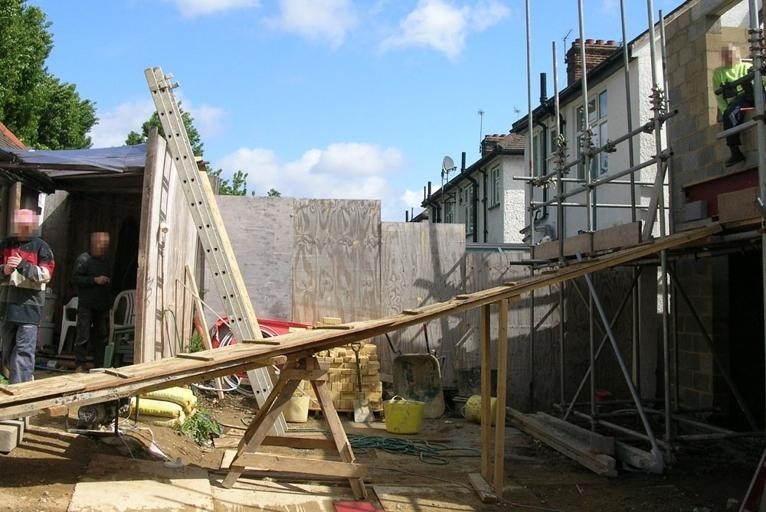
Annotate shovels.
[351,343,376,422]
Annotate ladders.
[146,67,288,437]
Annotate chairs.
[57,288,136,368]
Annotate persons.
[0,202,56,385]
[72,225,114,373]
[713,42,766,168]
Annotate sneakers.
[724,152,746,168]
